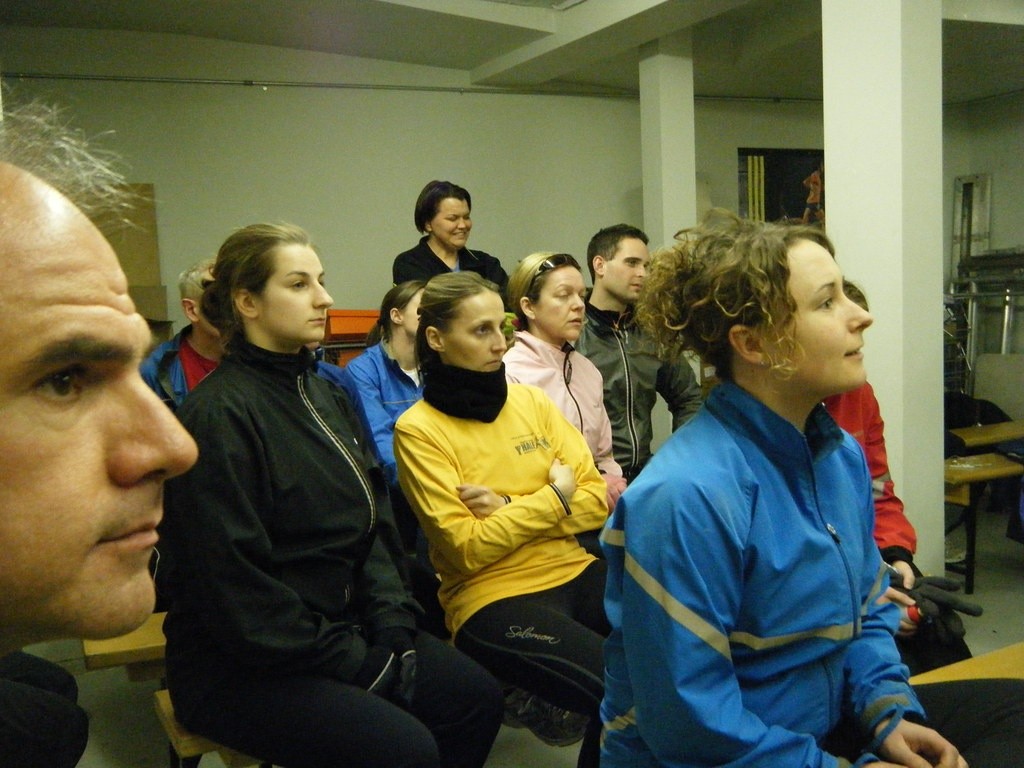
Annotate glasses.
[524,254,580,297]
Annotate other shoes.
[500,670,593,748]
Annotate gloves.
[368,648,418,703]
[882,562,984,638]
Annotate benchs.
[81,612,264,767]
[943,419,1024,595]
[907,639,1023,686]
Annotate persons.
[0,159,974,768]
[393,180,511,292]
[574,223,704,487]
[345,280,429,555]
[502,252,627,513]
